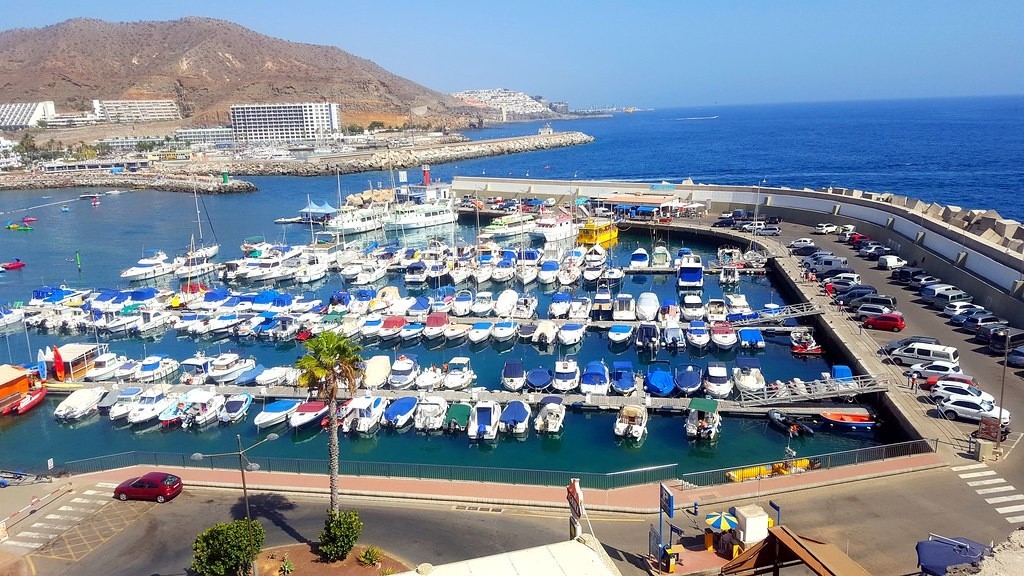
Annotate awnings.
[298,201,338,221]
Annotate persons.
[800,268,817,288]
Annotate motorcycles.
[971,428,1015,442]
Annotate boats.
[727,456,826,485]
[3,197,880,452]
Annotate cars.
[114,471,183,504]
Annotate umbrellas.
[705,510,738,553]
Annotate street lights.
[190,431,280,576]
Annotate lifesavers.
[614,427,622,435]
[632,430,640,437]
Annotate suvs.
[710,206,1024,425]
